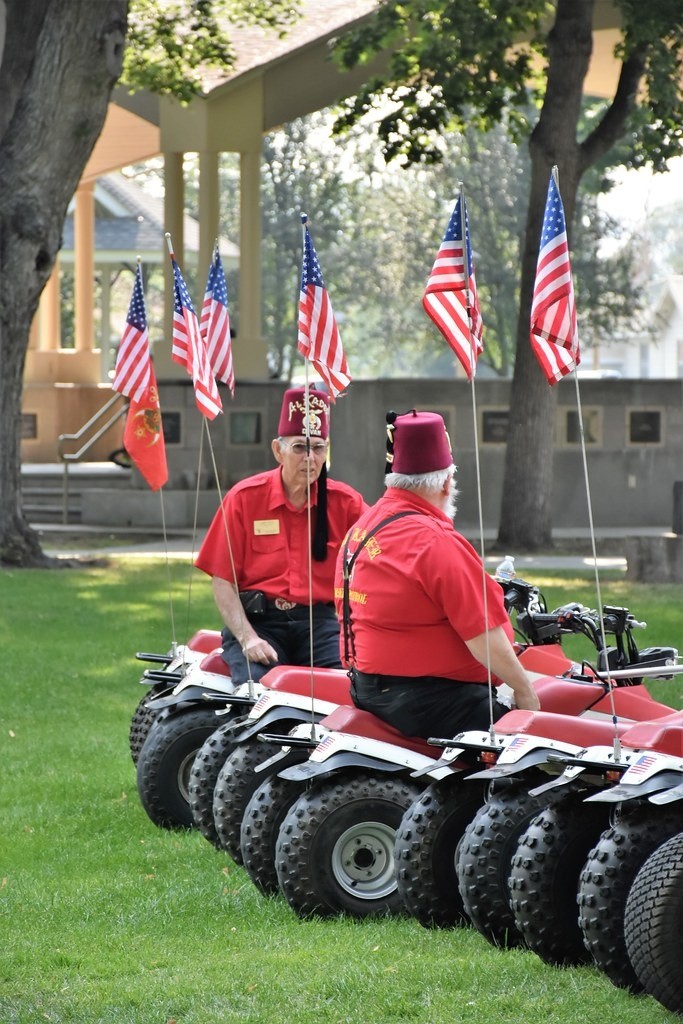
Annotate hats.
[278,382,331,561]
[382,408,453,474]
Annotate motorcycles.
[129,573,683,1015]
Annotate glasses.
[279,437,328,455]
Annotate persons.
[194,388,372,690]
[333,409,540,742]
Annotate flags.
[422,195,483,383]
[171,251,223,420]
[113,264,168,491]
[530,171,580,386]
[297,216,351,398]
[198,246,235,397]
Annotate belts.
[266,596,304,610]
